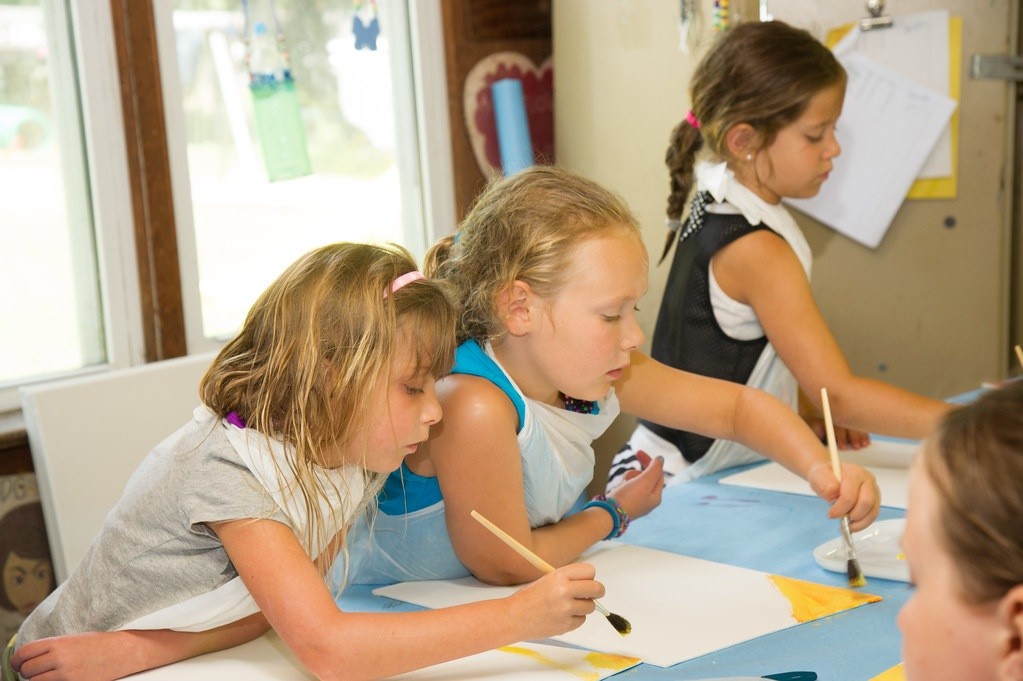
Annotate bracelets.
[583,495,630,541]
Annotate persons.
[608,21,960,493]
[0,242,603,681]
[323,166,881,593]
[897,379,1022,681]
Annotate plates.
[812,517,911,584]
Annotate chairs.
[21,351,224,584]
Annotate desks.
[110,385,1000,681]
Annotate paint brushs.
[469,510,632,637]
[820,386,867,588]
